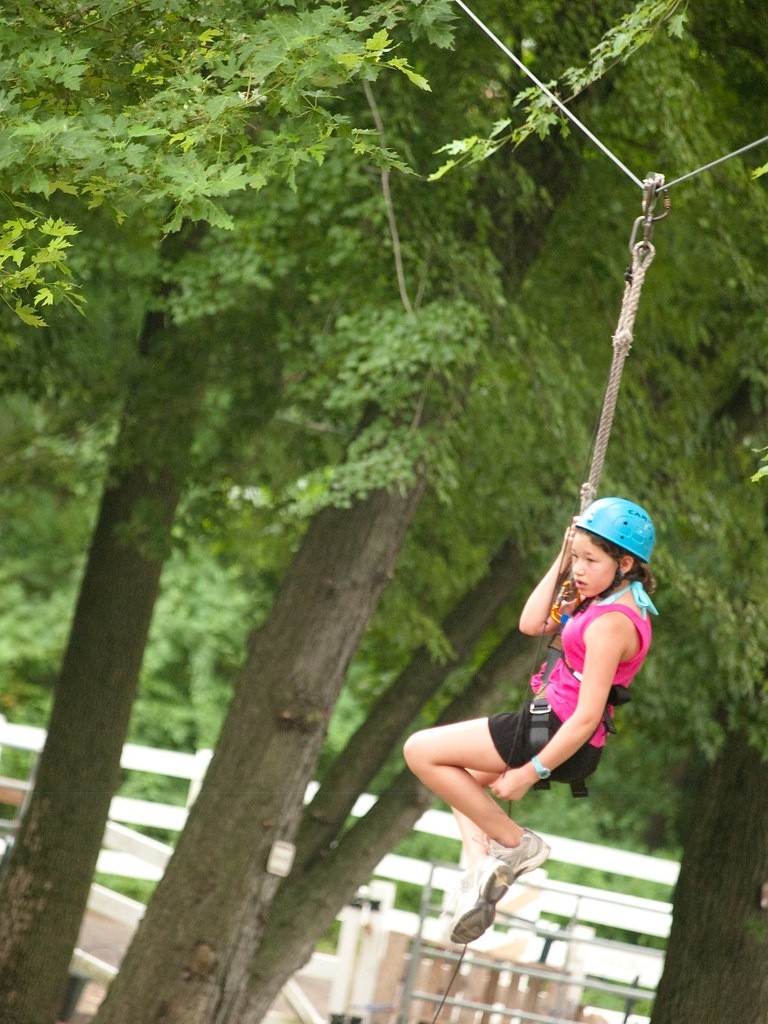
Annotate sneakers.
[488,825,550,878]
[450,858,514,945]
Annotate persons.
[402,496,658,944]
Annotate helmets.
[575,497,655,563]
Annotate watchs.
[532,756,551,779]
